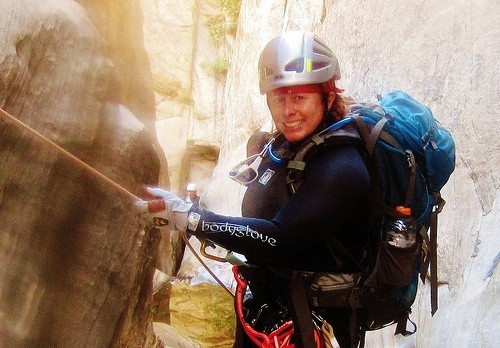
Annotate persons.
[134,31,380,348]
[182,183,201,260]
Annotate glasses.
[228,138,275,184]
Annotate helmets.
[258,31,340,94]
[187,184,197,191]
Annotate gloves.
[132,187,189,239]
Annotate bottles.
[388,207,417,247]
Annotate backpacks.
[247,92,457,331]
